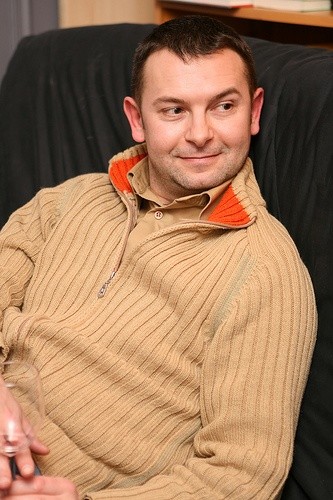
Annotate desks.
[153,0,333,50]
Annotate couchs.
[0,22,333,500]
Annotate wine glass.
[0,361,42,480]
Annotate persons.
[0,15,319,500]
